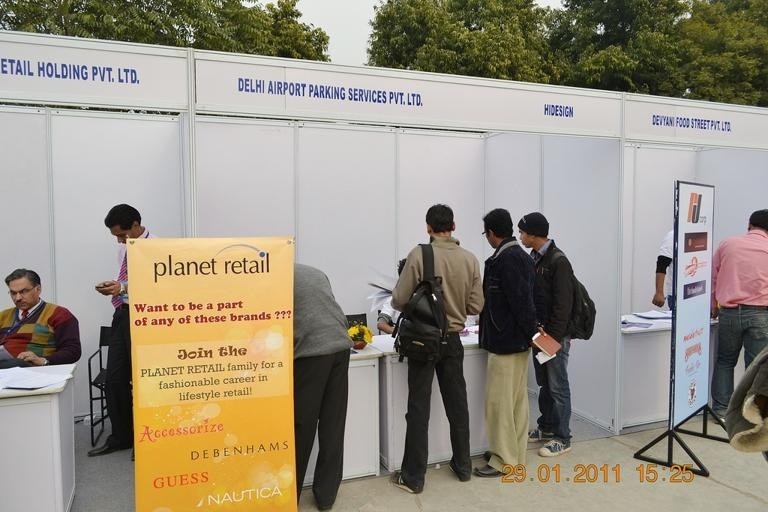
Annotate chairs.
[88,326,112,447]
[377,309,387,335]
[343,313,368,331]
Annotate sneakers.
[527,429,553,443]
[392,471,424,494]
[449,460,471,481]
[539,438,571,456]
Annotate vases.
[353,341,367,350]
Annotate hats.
[518,212,549,236]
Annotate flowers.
[347,322,374,345]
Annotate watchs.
[119,280,126,298]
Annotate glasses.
[481,231,488,238]
[8,287,35,296]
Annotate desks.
[295,343,383,488]
[1,365,77,512]
[620,308,717,432]
[368,328,490,474]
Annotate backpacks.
[547,252,596,341]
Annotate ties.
[111,256,127,310]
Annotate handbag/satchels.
[394,279,447,363]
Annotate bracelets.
[41,356,49,366]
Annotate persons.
[652,230,674,312]
[376,258,408,334]
[87,204,162,461]
[294,261,353,511]
[473,209,546,477]
[0,269,81,367]
[390,203,485,493]
[710,209,768,423]
[518,212,575,458]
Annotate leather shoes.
[474,464,501,478]
[483,451,491,462]
[87,444,118,456]
